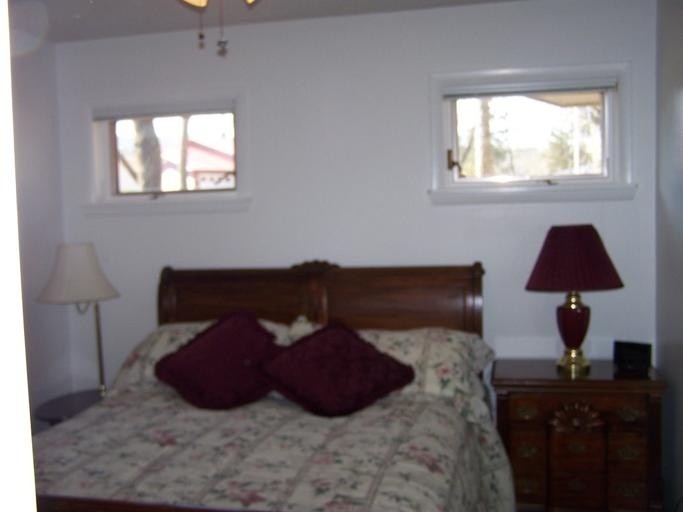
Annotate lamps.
[526,224,623,373]
[37,241,121,392]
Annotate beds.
[32,261,483,512]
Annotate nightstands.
[34,390,107,424]
[491,357,667,512]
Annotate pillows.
[287,315,492,415]
[152,312,281,412]
[258,316,415,417]
[111,319,287,402]
